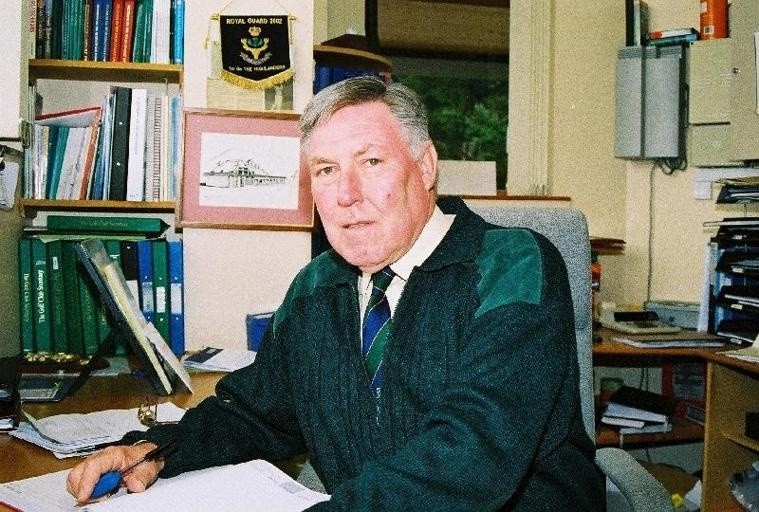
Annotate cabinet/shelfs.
[698,348,759,512]
[703,221,759,345]
[23,56,185,373]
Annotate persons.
[65,74,608,512]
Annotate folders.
[152,241,168,344]
[121,241,139,309]
[168,240,183,355]
[137,240,153,324]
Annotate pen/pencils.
[73,438,177,508]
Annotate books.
[644,27,698,45]
[601,384,680,437]
[25,0,184,63]
[26,86,183,201]
[24,205,195,397]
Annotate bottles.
[626,1,649,47]
[699,0,728,40]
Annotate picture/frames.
[174,108,315,233]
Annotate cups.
[599,376,624,409]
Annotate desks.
[1,371,331,512]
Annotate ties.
[362,266,395,399]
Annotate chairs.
[314,195,677,512]
